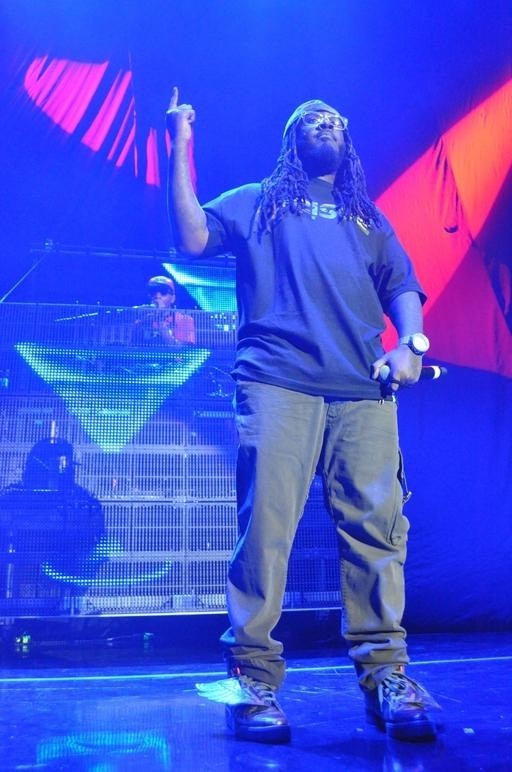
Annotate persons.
[132,276,197,362]
[164,87,444,742]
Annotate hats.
[281,100,341,139]
[148,276,175,294]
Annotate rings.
[390,377,404,386]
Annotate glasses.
[149,287,171,296]
[292,111,350,131]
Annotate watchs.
[397,331,431,356]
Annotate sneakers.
[364,667,438,740]
[224,675,291,742]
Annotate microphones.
[131,304,156,313]
[378,363,450,384]
